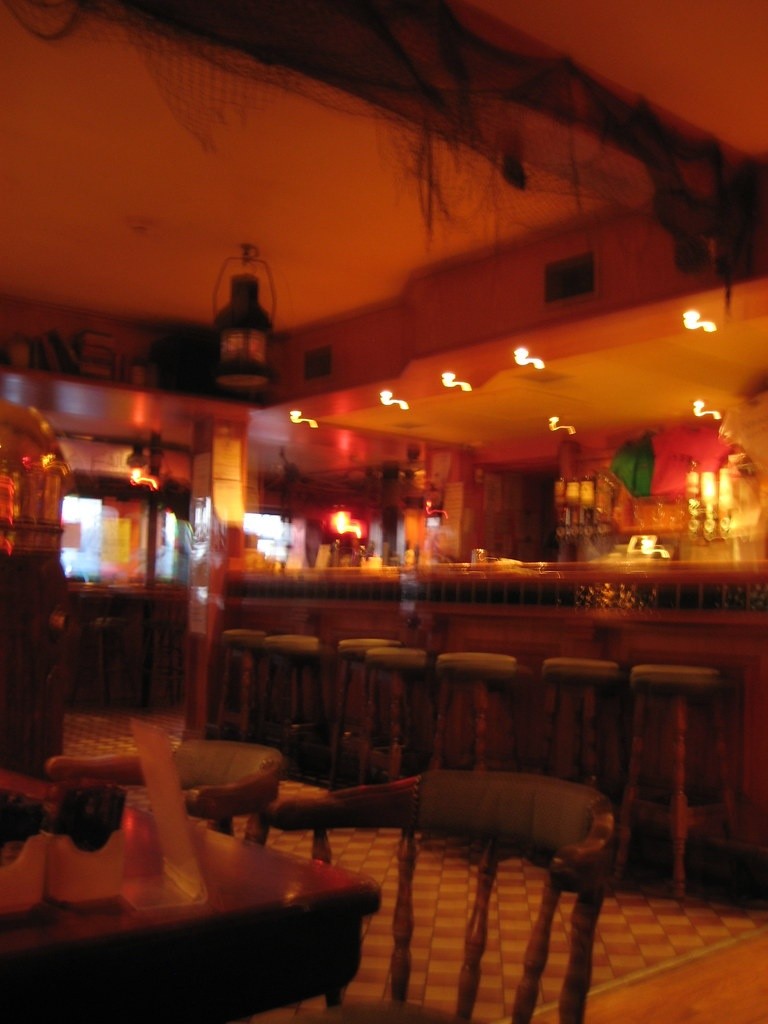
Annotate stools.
[421,651,516,855]
[613,665,740,900]
[328,639,402,789]
[257,634,328,784]
[538,657,620,789]
[215,629,267,742]
[68,614,185,713]
[359,648,428,784]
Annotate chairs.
[267,768,618,1024]
[44,739,284,846]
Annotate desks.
[0,768,381,1024]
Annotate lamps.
[214,243,280,395]
